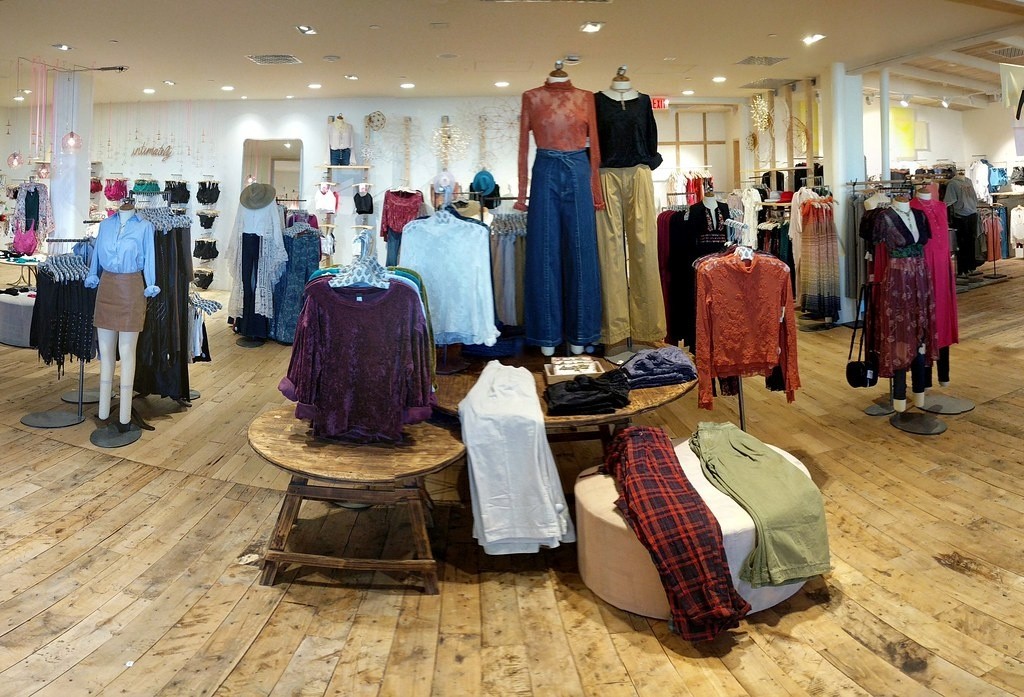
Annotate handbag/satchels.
[846,360,875,388]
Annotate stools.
[575,443,812,622]
[0,291,36,347]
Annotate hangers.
[185,288,223,314]
[661,167,839,273]
[38,174,222,282]
[286,178,528,289]
[24,175,38,186]
[971,156,1024,225]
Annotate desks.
[248,404,467,596]
[0,259,39,287]
[432,340,700,454]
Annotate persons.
[84,204,161,432]
[872,187,959,413]
[944,172,978,275]
[513,61,606,358]
[224,183,289,340]
[585,65,668,344]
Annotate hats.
[240,183,276,209]
[434,172,455,193]
[472,171,495,196]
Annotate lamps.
[969,97,976,106]
[899,94,914,107]
[942,97,952,109]
[35,69,50,179]
[62,63,82,153]
[7,57,23,169]
